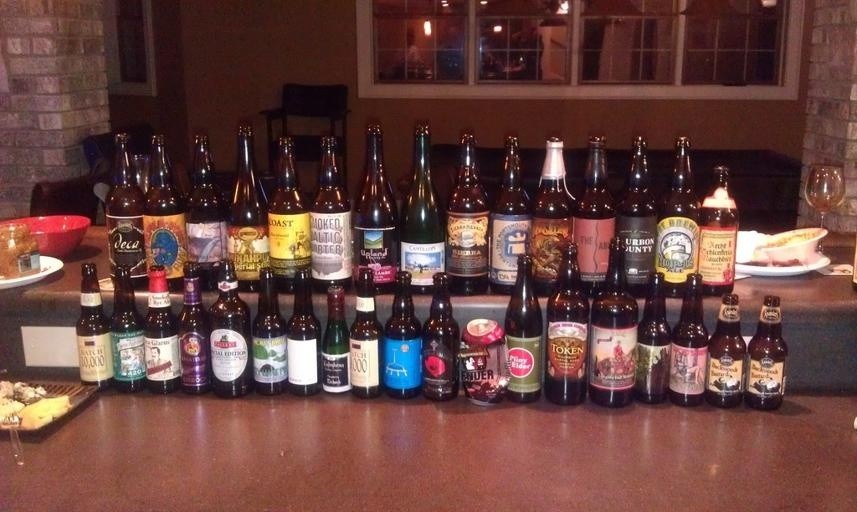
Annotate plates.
[734,253,830,278]
[0,256,65,289]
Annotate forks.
[3,412,25,469]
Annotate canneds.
[458,318,514,408]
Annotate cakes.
[0,379,69,431]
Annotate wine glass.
[804,163,843,255]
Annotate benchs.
[395,142,798,233]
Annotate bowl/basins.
[754,225,828,263]
[0,215,92,259]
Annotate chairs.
[258,83,350,186]
[26,178,101,225]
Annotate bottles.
[107,134,742,291]
[75,236,786,409]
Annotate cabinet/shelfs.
[104,1,179,99]
